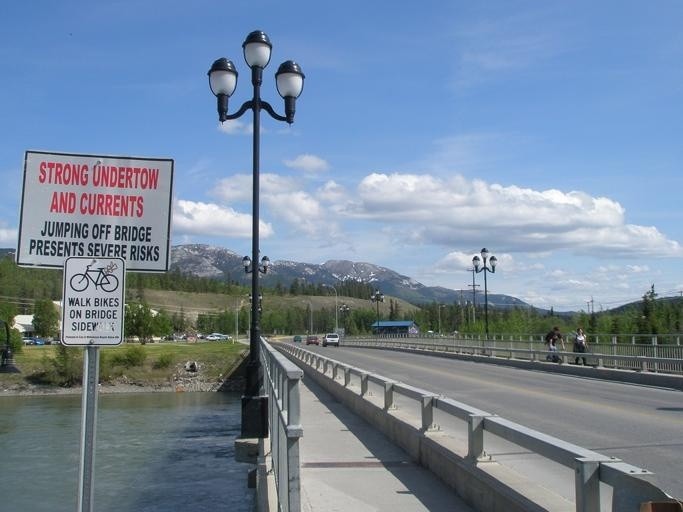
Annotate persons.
[546,326,566,362]
[572,328,589,366]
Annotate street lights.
[472,248,496,340]
[370,289,383,334]
[206,31,304,438]
[339,303,350,335]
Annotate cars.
[22,337,46,345]
[306,336,318,345]
[322,333,339,347]
[161,332,229,342]
[293,336,301,342]
